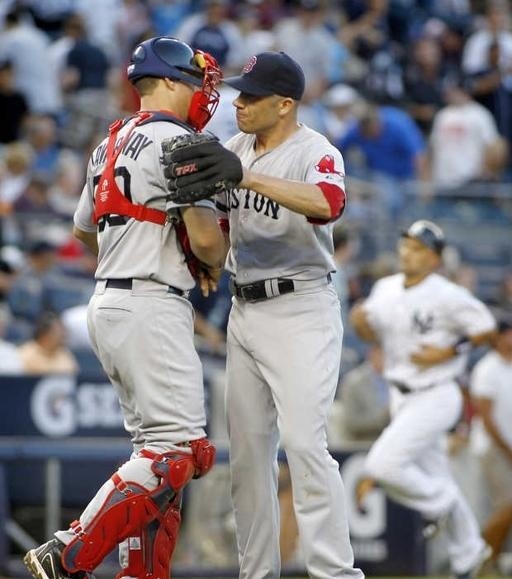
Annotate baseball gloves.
[159,131,243,204]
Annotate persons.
[467,309,511,578]
[0,0,512,451]
[352,217,496,579]
[171,49,363,579]
[22,38,230,579]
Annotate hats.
[220,52,304,100]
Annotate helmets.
[400,220,444,256]
[128,37,224,132]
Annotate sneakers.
[24,539,94,578]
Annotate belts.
[228,276,293,304]
[394,382,411,393]
[104,279,185,295]
